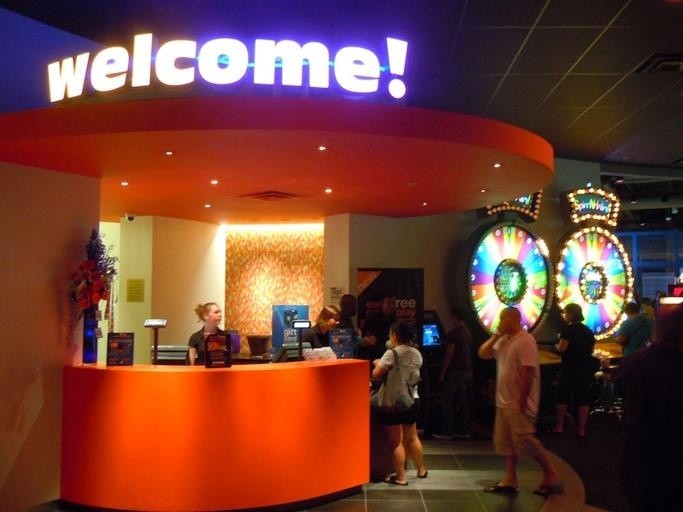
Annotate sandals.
[534,482,566,497]
[482,482,519,494]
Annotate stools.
[593,364,626,423]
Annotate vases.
[82,303,98,364]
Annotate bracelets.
[492,330,500,339]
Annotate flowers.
[68,227,120,339]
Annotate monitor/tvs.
[151,346,191,365]
[422,323,441,346]
[272,342,312,363]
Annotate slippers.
[418,470,429,480]
[382,473,410,486]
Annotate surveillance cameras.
[126,214,135,221]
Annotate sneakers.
[433,431,473,441]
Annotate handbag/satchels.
[377,349,412,411]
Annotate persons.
[478,305,564,495]
[337,293,360,350]
[305,304,338,349]
[545,303,597,438]
[186,303,231,365]
[371,322,427,485]
[363,296,397,358]
[613,290,667,358]
[431,305,475,441]
